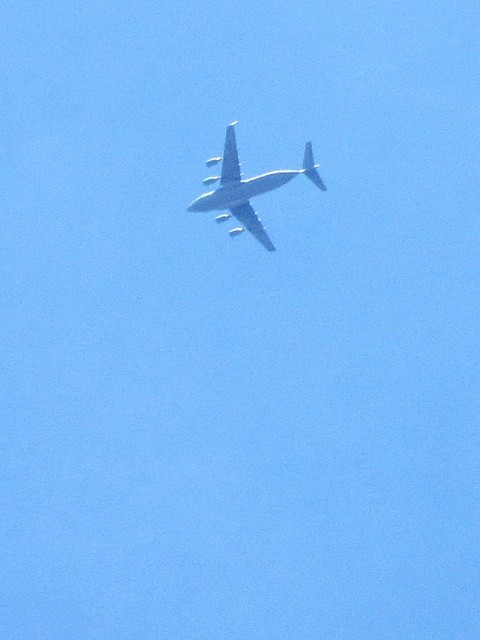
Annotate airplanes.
[187,119,331,253]
